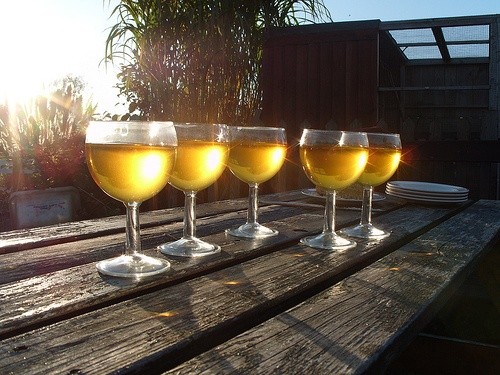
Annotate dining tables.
[1,187,500,375]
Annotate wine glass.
[225,126,287,238]
[157,122,231,258]
[299,128,369,251]
[340,133,402,240]
[85,121,178,278]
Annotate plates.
[302,189,386,202]
[384,181,470,203]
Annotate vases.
[9,186,82,228]
